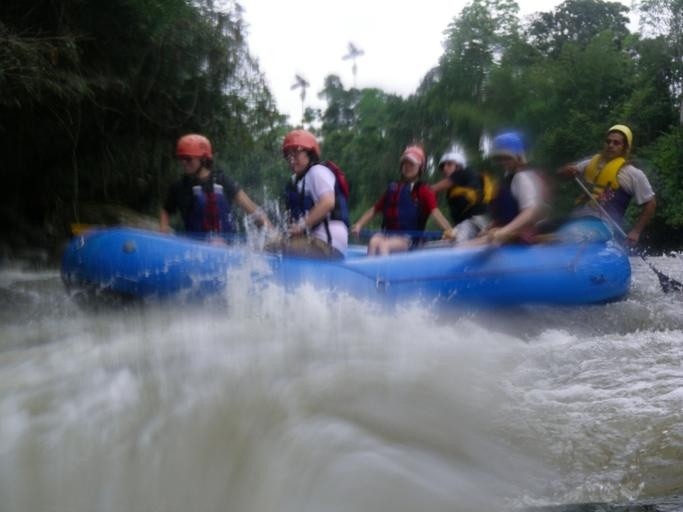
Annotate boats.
[59,215,632,325]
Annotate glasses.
[604,138,620,145]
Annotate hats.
[399,146,423,165]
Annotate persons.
[462,133,553,248]
[160,134,280,248]
[431,151,494,242]
[349,145,460,258]
[553,123,656,248]
[260,129,352,258]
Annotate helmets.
[607,124,632,147]
[483,132,525,161]
[283,130,318,156]
[175,134,211,160]
[437,153,466,171]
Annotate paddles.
[574,176,683,296]
